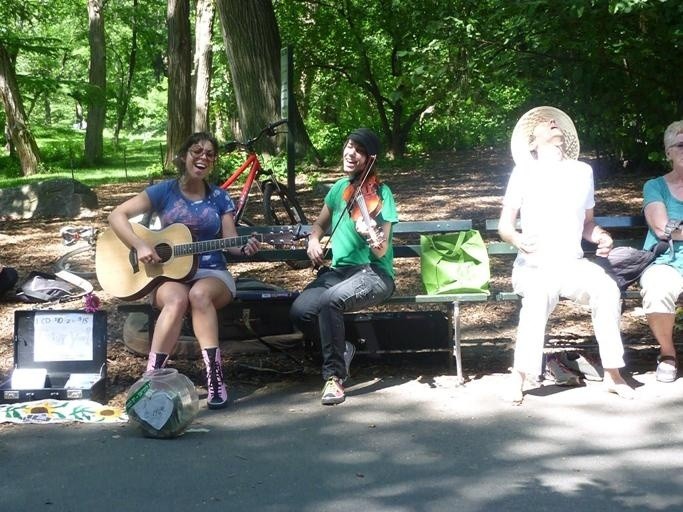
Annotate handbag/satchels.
[419,229,490,296]
[592,218,681,291]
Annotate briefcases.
[1,309,107,404]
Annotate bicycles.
[217,117,312,271]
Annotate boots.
[147,351,169,372]
[202,346,228,409]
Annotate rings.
[147,257,151,262]
[144,261,148,264]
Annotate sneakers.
[541,353,580,386]
[321,375,346,404]
[343,341,357,385]
[556,350,603,381]
[654,354,678,382]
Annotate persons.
[497,116,639,408]
[638,119,683,383]
[287,127,400,406]
[107,131,262,409]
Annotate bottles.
[123,367,202,439]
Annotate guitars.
[95,222,294,300]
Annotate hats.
[349,127,379,153]
[510,106,580,164]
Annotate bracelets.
[598,231,610,237]
[239,244,246,257]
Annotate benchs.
[487,214,681,381]
[116,216,492,386]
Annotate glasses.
[669,142,682,147]
[188,144,219,161]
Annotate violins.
[343,175,386,249]
